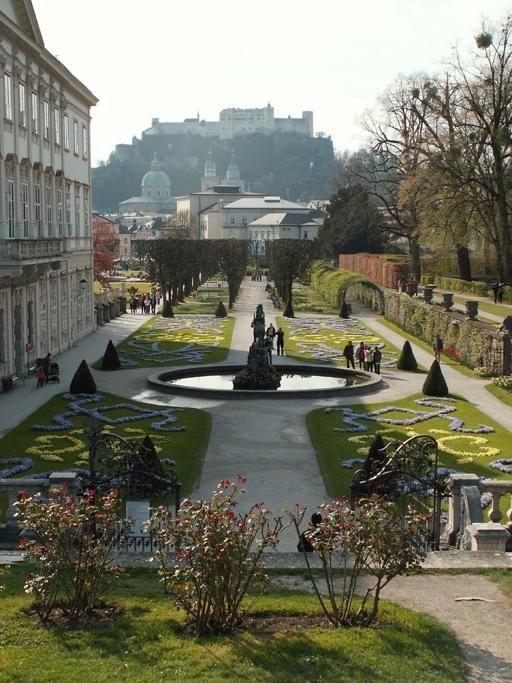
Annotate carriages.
[128,292,145,314]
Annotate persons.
[272,326,285,355]
[433,335,443,362]
[36,351,59,388]
[492,281,499,303]
[297,512,326,552]
[266,322,275,350]
[129,288,162,313]
[265,337,276,364]
[343,340,382,374]
[396,272,419,298]
[497,284,504,303]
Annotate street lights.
[151,286,157,315]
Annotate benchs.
[2,373,26,391]
[27,359,40,377]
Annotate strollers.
[46,362,62,384]
